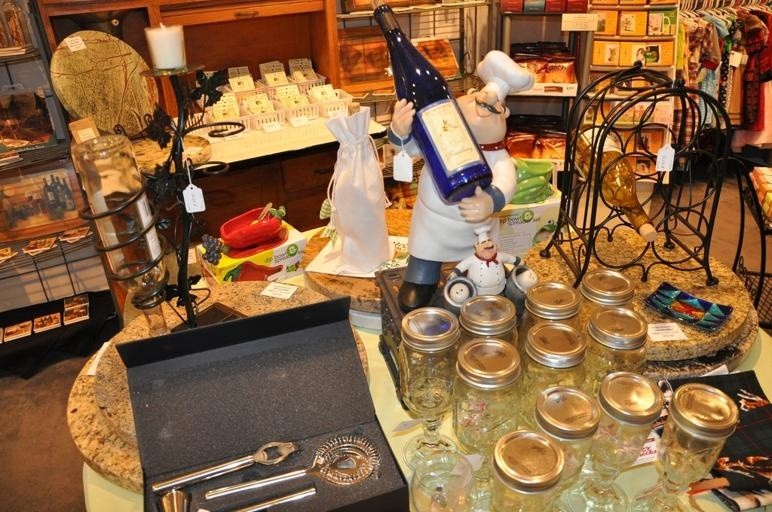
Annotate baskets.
[270,93,319,124]
[242,98,288,130]
[172,113,217,139]
[226,81,266,106]
[197,87,234,108]
[288,71,327,95]
[308,88,354,120]
[206,103,253,134]
[256,76,300,100]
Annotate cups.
[412,450,479,511]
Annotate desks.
[82,219,772,511]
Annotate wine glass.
[521,320,588,425]
[398,306,459,468]
[519,282,580,355]
[629,383,738,512]
[586,306,648,390]
[532,387,600,496]
[567,372,662,512]
[490,429,563,512]
[456,294,519,347]
[451,337,522,453]
[579,268,633,335]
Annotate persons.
[387,51,534,313]
[446,225,527,297]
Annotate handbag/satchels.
[317,105,393,273]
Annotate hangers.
[678,0,772,42]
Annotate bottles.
[569,125,656,242]
[41,174,74,212]
[72,135,171,340]
[371,1,493,206]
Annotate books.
[625,156,660,181]
[1,246,14,261]
[585,100,671,125]
[592,40,675,67]
[59,231,95,244]
[62,226,91,242]
[23,244,60,256]
[608,129,664,154]
[593,8,676,36]
[24,237,56,251]
[589,72,668,95]
[591,0,679,6]
[1,252,20,266]
[0,293,89,344]
